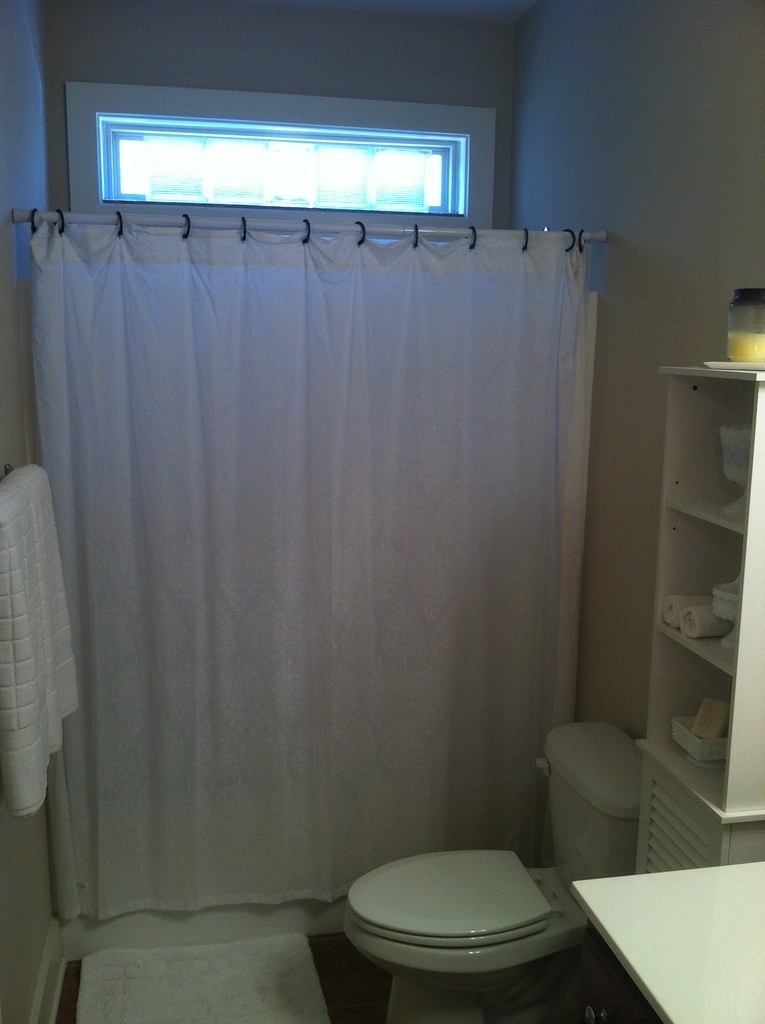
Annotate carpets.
[75,932,333,1024]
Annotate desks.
[571,861,765,1024]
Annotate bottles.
[727,289,765,361]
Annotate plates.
[702,361,764,370]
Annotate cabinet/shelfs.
[633,366,765,874]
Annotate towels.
[663,594,736,638]
[0,463,79,821]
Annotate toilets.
[342,722,643,1023]
